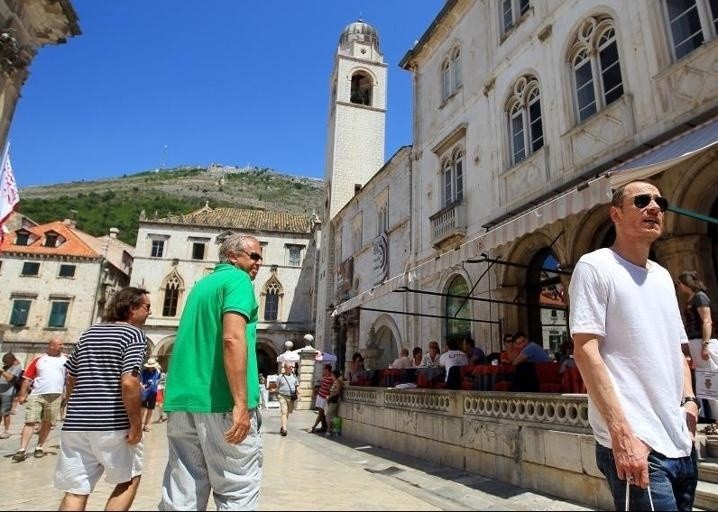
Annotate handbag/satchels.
[289,392,298,400]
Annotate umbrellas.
[277,345,337,362]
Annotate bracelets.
[702,341,709,346]
[681,397,701,412]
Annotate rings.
[626,475,634,482]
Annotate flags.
[0,153,20,253]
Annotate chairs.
[350,360,587,394]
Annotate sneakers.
[12,450,27,461]
[280,426,337,437]
[0,431,12,439]
[34,446,44,458]
[143,415,167,432]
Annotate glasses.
[139,303,151,312]
[235,249,262,262]
[616,194,669,213]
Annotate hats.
[143,358,159,368]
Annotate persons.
[0,338,269,461]
[348,332,576,392]
[324,371,342,436]
[53,287,152,512]
[308,365,345,432]
[157,233,264,512]
[568,179,699,511]
[277,363,299,436]
[678,271,718,424]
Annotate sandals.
[701,424,718,435]
